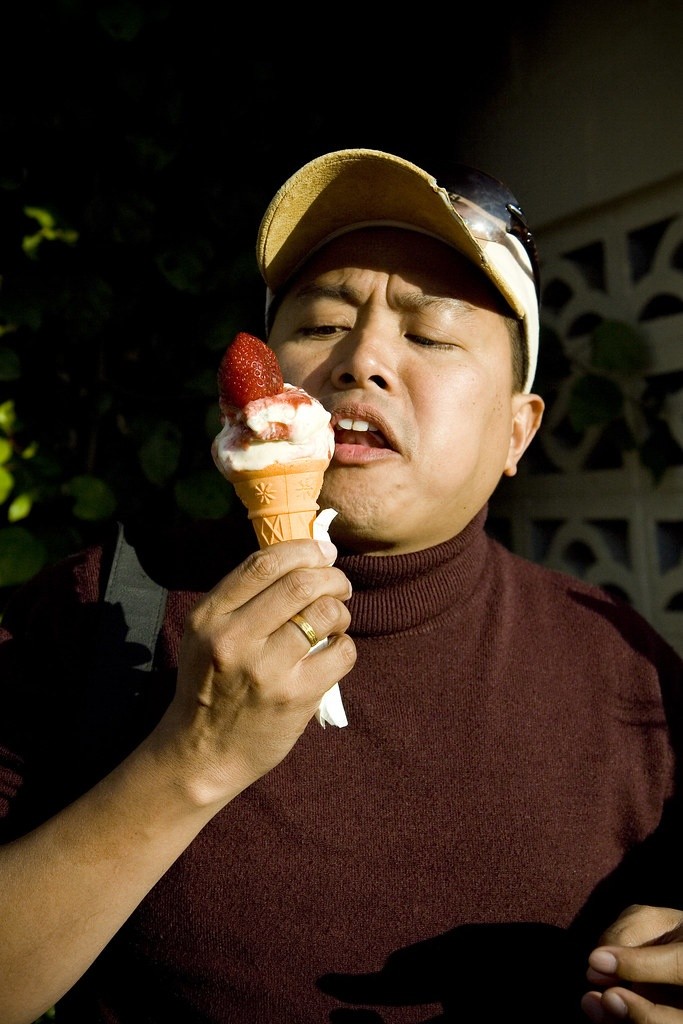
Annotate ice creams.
[208,380,335,550]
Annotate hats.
[257,149,540,396]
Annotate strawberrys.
[215,332,285,412]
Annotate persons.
[0,148,682,1024]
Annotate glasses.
[434,166,542,318]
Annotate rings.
[291,614,318,647]
